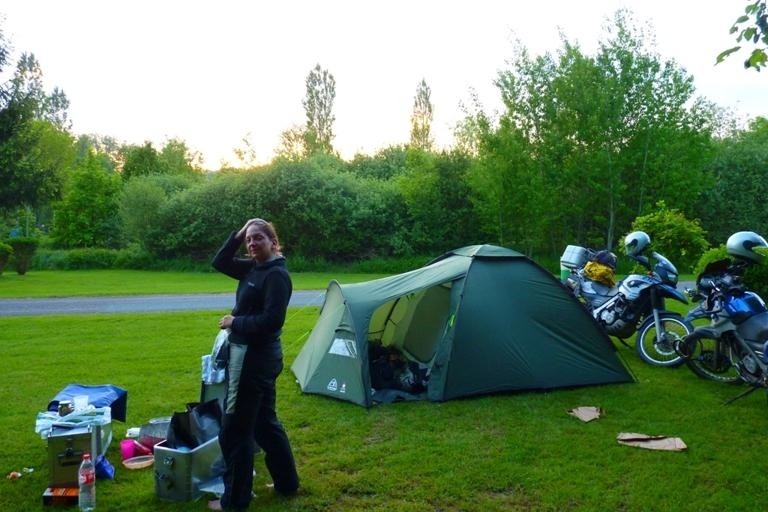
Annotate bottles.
[78,453,95,512]
[134,440,152,456]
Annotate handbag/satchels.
[584,260,616,287]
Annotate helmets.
[726,231,768,266]
[624,231,651,255]
[598,251,617,268]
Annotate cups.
[73,395,88,412]
[120,439,135,460]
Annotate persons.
[208,218,299,510]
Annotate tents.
[291,244,639,408]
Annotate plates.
[122,454,154,470]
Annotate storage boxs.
[153,435,226,501]
[47,406,113,486]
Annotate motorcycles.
[678,249,768,410]
[559,236,696,371]
